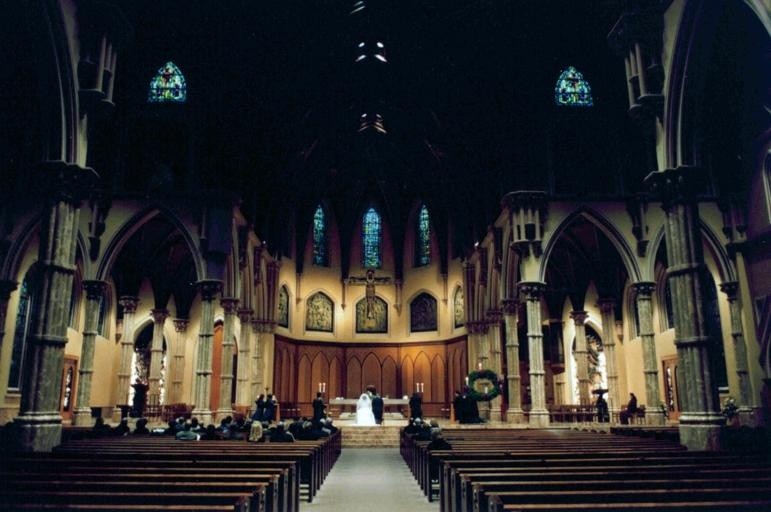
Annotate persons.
[131,378,148,415]
[356,393,375,425]
[372,394,384,424]
[264,394,276,420]
[460,386,479,423]
[312,392,326,419]
[453,391,463,421]
[91,415,337,442]
[597,394,608,423]
[628,392,637,414]
[404,392,452,451]
[252,394,266,421]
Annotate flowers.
[462,366,504,400]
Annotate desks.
[329,397,411,421]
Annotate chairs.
[548,403,646,424]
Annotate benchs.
[399,426,771,511]
[1,406,344,511]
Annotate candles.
[420,382,423,393]
[319,383,320,392]
[416,382,419,392]
[478,362,481,370]
[465,376,468,385]
[484,386,487,394]
[322,382,324,393]
